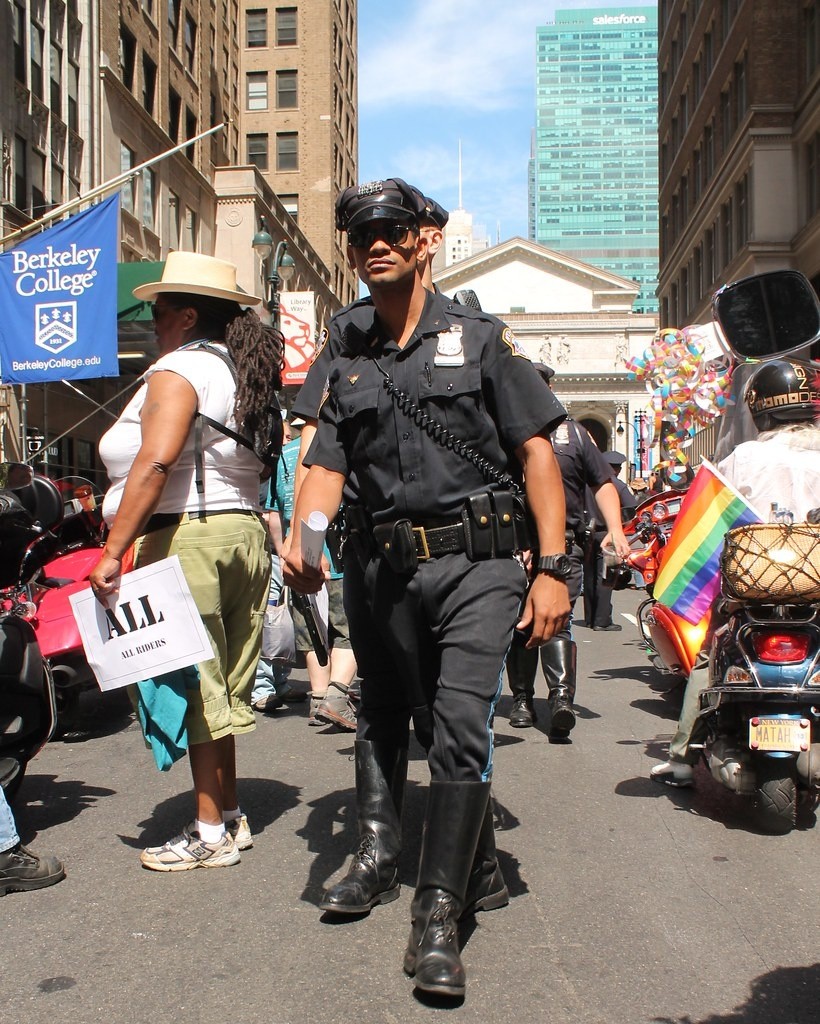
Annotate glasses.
[347,225,419,248]
[151,303,191,320]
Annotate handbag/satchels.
[263,585,307,666]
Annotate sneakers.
[141,825,241,871]
[308,694,358,730]
[183,814,254,849]
[0,841,65,897]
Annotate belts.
[136,509,251,534]
[349,522,464,560]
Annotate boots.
[403,780,491,997]
[540,639,576,743]
[319,732,411,911]
[506,627,539,727]
[460,792,508,920]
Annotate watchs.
[537,552,572,575]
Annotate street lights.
[251,213,297,331]
[616,408,646,478]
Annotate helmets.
[744,358,820,432]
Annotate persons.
[0,786,64,896]
[512,363,629,741]
[583,450,636,630]
[650,471,665,493]
[251,419,357,729]
[649,361,820,787]
[281,179,572,992]
[90,251,286,872]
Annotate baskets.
[723,525,820,604]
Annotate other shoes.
[586,622,593,628]
[650,759,694,787]
[253,695,282,711]
[593,624,622,631]
[276,688,306,702]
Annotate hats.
[602,451,626,468]
[533,363,554,386]
[132,250,262,305]
[423,196,449,230]
[334,178,426,233]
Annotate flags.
[653,464,759,626]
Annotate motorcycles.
[0,460,135,802]
[613,487,819,835]
